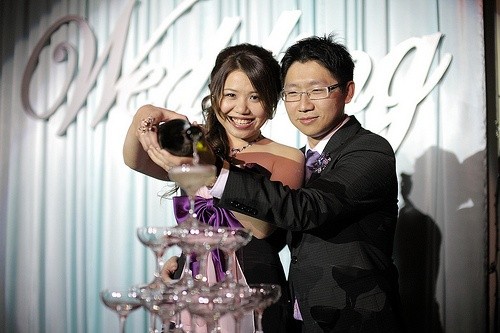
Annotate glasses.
[280,82,344,102]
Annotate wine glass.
[169,226,253,291]
[138,282,264,333]
[251,283,282,333]
[167,164,217,228]
[132,227,180,297]
[98,290,145,333]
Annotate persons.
[191,29,407,333]
[123,42,305,333]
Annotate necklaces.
[230,131,265,158]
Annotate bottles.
[156,118,204,158]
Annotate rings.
[133,116,157,135]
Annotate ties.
[306,149,320,185]
